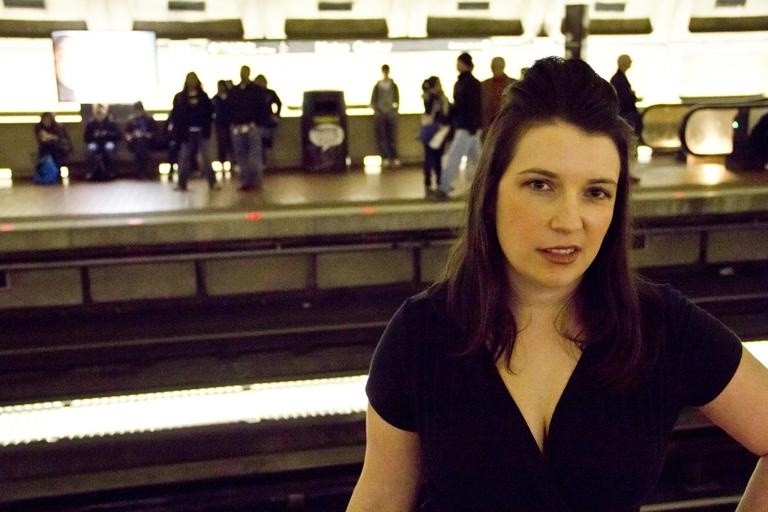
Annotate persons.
[609,55,647,145]
[33,66,282,191]
[346,55,768,511]
[419,48,533,197]
[370,64,400,169]
